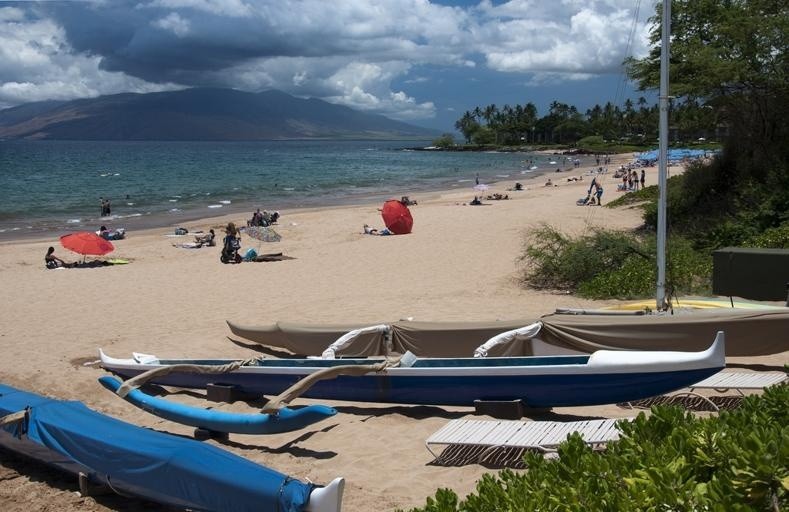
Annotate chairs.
[627,370,788,411]
[425,417,635,466]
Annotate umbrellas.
[382,199,413,234]
[60,231,114,263]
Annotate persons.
[364,224,392,235]
[101,202,105,216]
[99,225,108,241]
[246,209,280,228]
[104,200,111,216]
[44,246,64,270]
[469,155,646,206]
[221,230,237,263]
[194,229,215,243]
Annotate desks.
[711,247,789,308]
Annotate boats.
[1,384,347,512]
[225,300,788,358]
[79,329,728,409]
